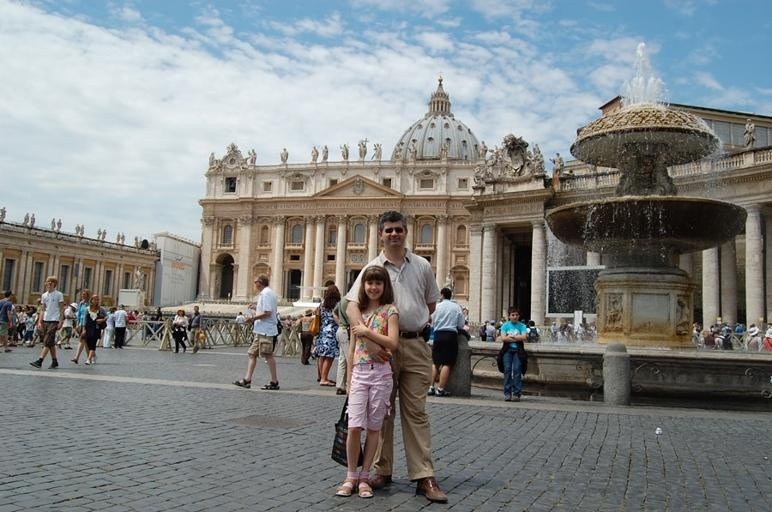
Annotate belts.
[400,329,424,339]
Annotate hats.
[721,325,744,336]
[304,310,313,315]
[748,326,759,337]
[195,329,205,341]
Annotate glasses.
[383,227,404,234]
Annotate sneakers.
[434,388,451,397]
[427,386,434,395]
[29,361,42,367]
[71,354,97,366]
[512,395,521,401]
[172,346,200,353]
[317,378,336,386]
[504,394,511,402]
[48,361,59,369]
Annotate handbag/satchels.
[98,313,106,328]
[295,322,301,334]
[308,307,321,336]
[331,393,364,469]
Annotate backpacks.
[527,328,540,344]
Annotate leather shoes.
[415,476,448,502]
[369,472,393,488]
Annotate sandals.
[358,477,374,498]
[260,381,279,390]
[336,477,356,496]
[232,378,251,388]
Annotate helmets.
[69,301,77,310]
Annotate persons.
[394,143,417,162]
[116,230,125,245]
[479,315,597,342]
[692,321,772,353]
[135,235,157,252]
[233,272,281,391]
[0,275,203,369]
[208,153,216,167]
[97,226,107,241]
[51,218,62,232]
[373,143,382,160]
[744,118,756,148]
[499,306,529,402]
[285,308,316,364]
[530,145,564,176]
[23,211,36,228]
[313,285,341,387]
[311,144,329,162]
[341,143,349,160]
[280,147,288,163]
[358,143,367,158]
[439,141,487,159]
[0,207,7,221]
[248,149,257,164]
[236,311,246,329]
[426,287,465,396]
[345,210,449,503]
[75,223,85,236]
[334,265,400,497]
